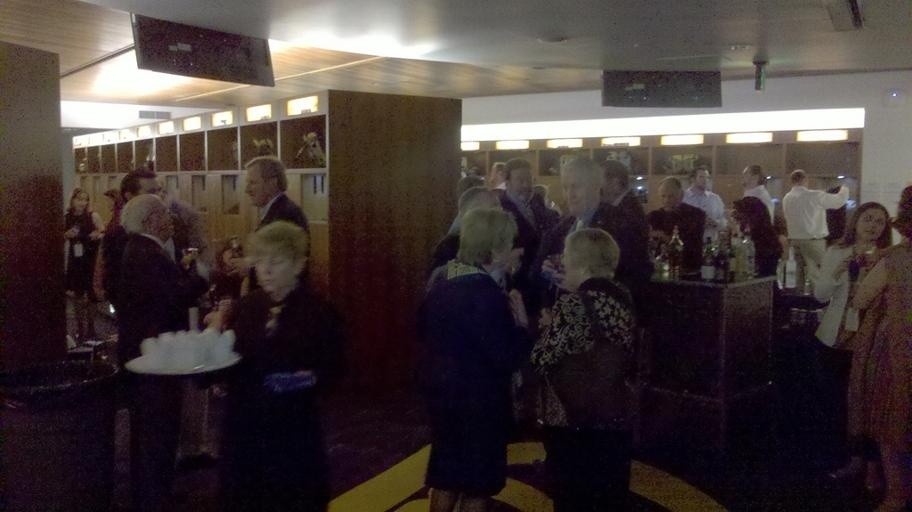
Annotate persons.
[420,207,531,512]
[65,155,344,512]
[741,163,774,225]
[430,158,650,311]
[646,177,706,269]
[530,228,636,512]
[783,169,850,293]
[733,195,783,296]
[813,201,891,493]
[847,187,911,512]
[682,165,726,243]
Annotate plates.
[124,349,243,377]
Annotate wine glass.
[72,225,81,239]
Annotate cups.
[96,223,104,233]
[548,253,561,272]
[852,233,866,253]
[865,249,879,271]
[141,328,237,368]
[182,247,198,260]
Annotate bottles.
[699,220,756,282]
[197,282,218,309]
[229,235,245,259]
[645,223,685,272]
[775,241,814,298]
[790,305,826,326]
[670,152,699,175]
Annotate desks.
[630,266,778,456]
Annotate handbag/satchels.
[586,348,625,423]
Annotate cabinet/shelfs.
[462,147,864,207]
[70,88,463,370]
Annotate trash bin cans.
[0,360,119,512]
[122,348,243,512]
